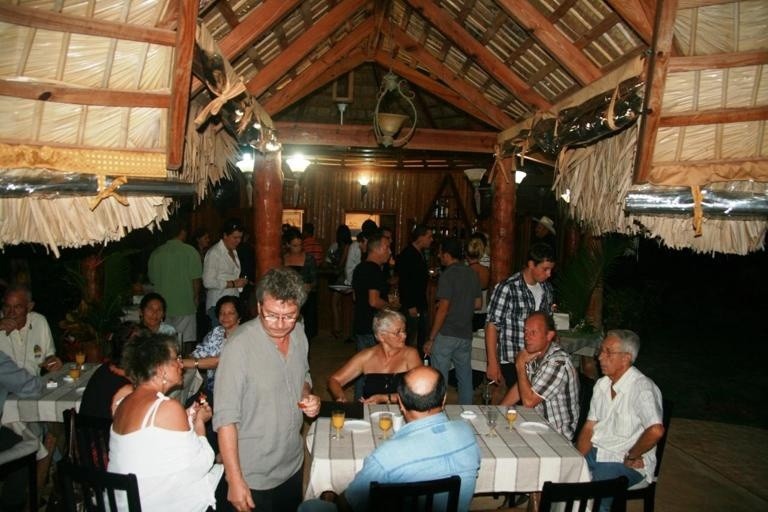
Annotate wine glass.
[392,414,403,433]
[331,409,346,441]
[484,411,501,440]
[482,383,492,410]
[506,404,518,432]
[377,412,393,440]
[75,350,86,370]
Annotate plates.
[76,387,85,396]
[519,421,549,434]
[370,411,394,423]
[342,420,373,432]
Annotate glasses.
[383,328,407,337]
[257,301,302,323]
[596,346,631,358]
[167,354,183,364]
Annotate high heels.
[330,327,355,346]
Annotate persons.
[297,366,481,510]
[211,267,323,512]
[90,332,226,510]
[575,328,667,512]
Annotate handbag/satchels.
[42,453,88,512]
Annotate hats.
[531,214,558,236]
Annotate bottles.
[432,197,450,217]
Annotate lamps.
[358,178,369,201]
[235,151,254,209]
[514,170,526,199]
[463,167,492,216]
[370,20,417,151]
[282,153,312,208]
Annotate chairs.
[175,332,184,357]
[353,369,367,405]
[369,476,461,512]
[63,408,113,470]
[57,459,143,512]
[628,394,672,512]
[538,476,627,512]
[2,426,39,512]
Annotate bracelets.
[625,453,643,460]
